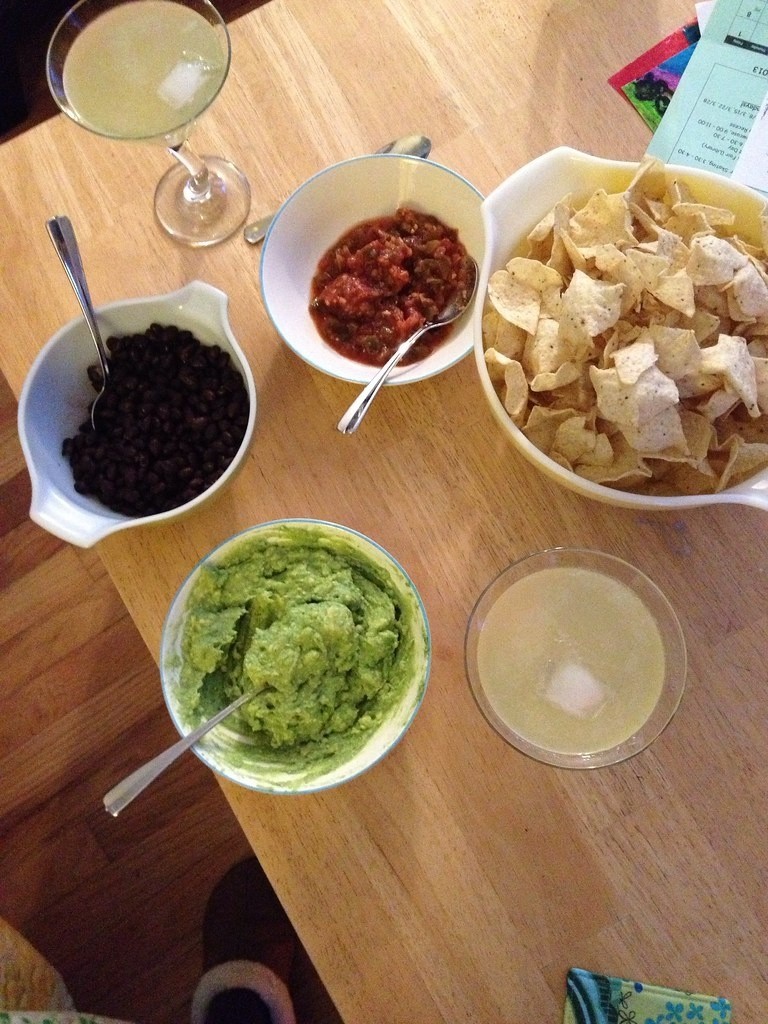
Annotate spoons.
[46,216,111,430]
[243,134,431,244]
[336,257,478,436]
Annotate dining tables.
[0,0,767,1024]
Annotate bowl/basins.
[472,147,768,509]
[17,280,257,549]
[260,153,486,387]
[159,517,431,792]
[463,545,691,771]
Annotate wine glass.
[45,0,249,249]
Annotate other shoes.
[188,858,301,1024]
